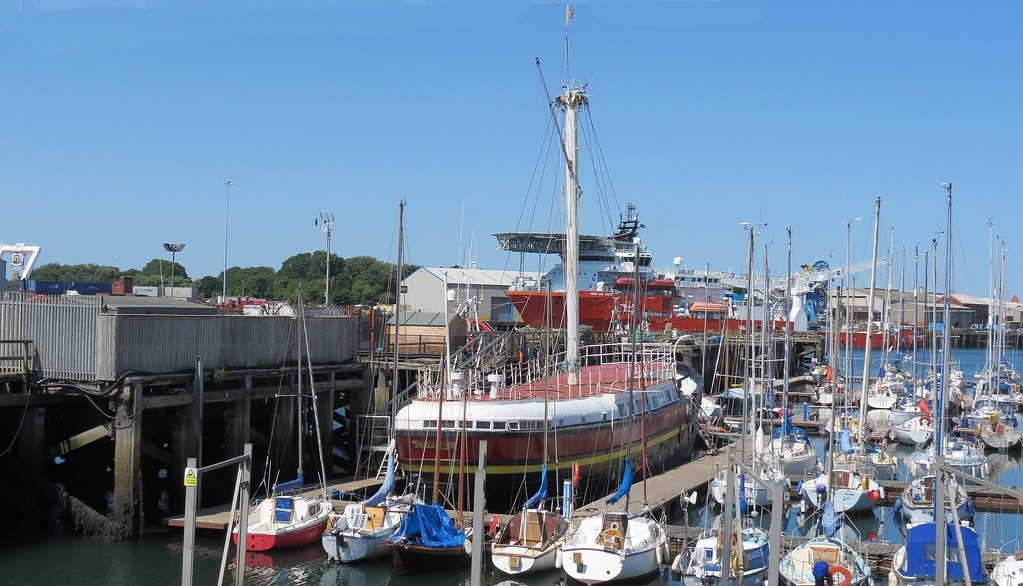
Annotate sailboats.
[486,176,1022,586]
[385,4,704,511]
[383,335,507,574]
[227,282,332,555]
[318,196,431,564]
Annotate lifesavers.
[825,566,851,586]
[598,527,624,551]
[778,407,791,418]
[732,550,748,577]
[489,514,500,538]
[573,461,581,488]
[919,415,932,427]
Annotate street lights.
[221,178,234,303]
[160,242,186,298]
